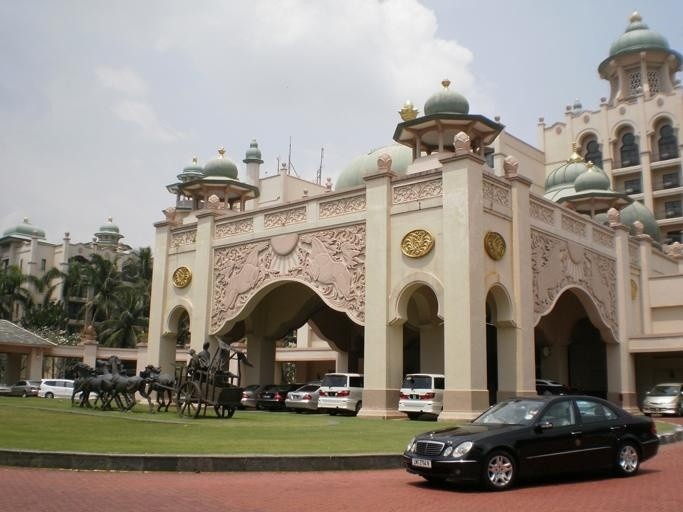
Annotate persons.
[186,349,201,381]
[198,342,210,370]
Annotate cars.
[536,379,571,395]
[643,382,683,417]
[402,395,659,492]
[238,384,321,413]
[1,380,99,399]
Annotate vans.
[399,374,444,419]
[318,373,364,415]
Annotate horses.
[65,355,178,413]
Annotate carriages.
[65,336,243,419]
